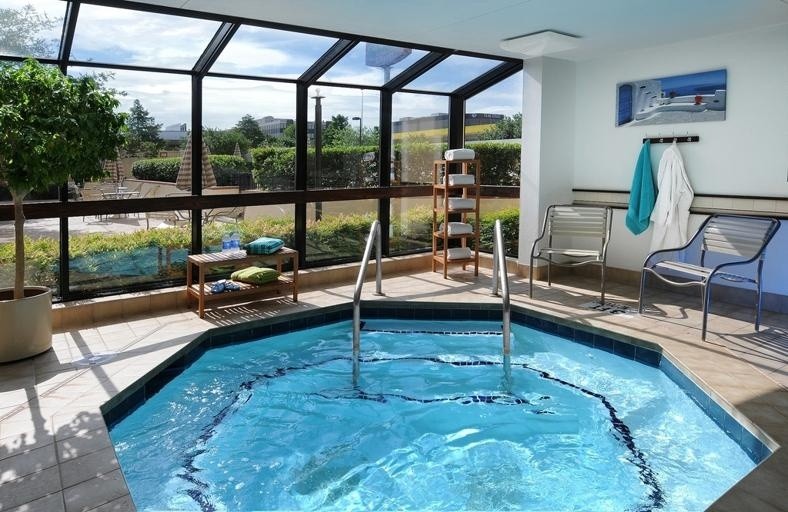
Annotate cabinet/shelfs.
[185,244,299,319]
[429,158,481,280]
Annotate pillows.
[227,264,283,284]
[241,236,287,256]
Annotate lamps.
[498,27,586,61]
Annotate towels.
[646,138,696,270]
[441,247,473,260]
[441,173,476,187]
[442,148,477,162]
[622,138,660,237]
[442,197,477,211]
[438,221,473,235]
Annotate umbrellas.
[233,142,243,157]
[175,132,218,199]
[99,143,127,185]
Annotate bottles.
[221,229,240,255]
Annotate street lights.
[352,116,362,147]
[309,87,326,222]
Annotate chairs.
[68,180,249,227]
[634,210,782,343]
[526,203,614,305]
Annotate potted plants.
[0,45,137,365]
